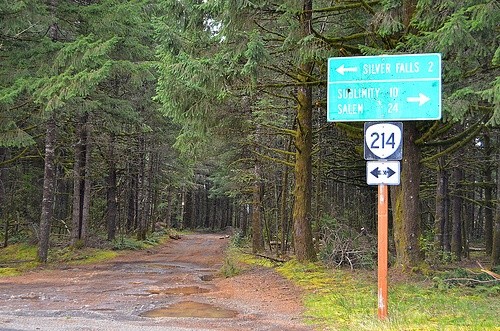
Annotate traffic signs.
[326,53,442,122]
